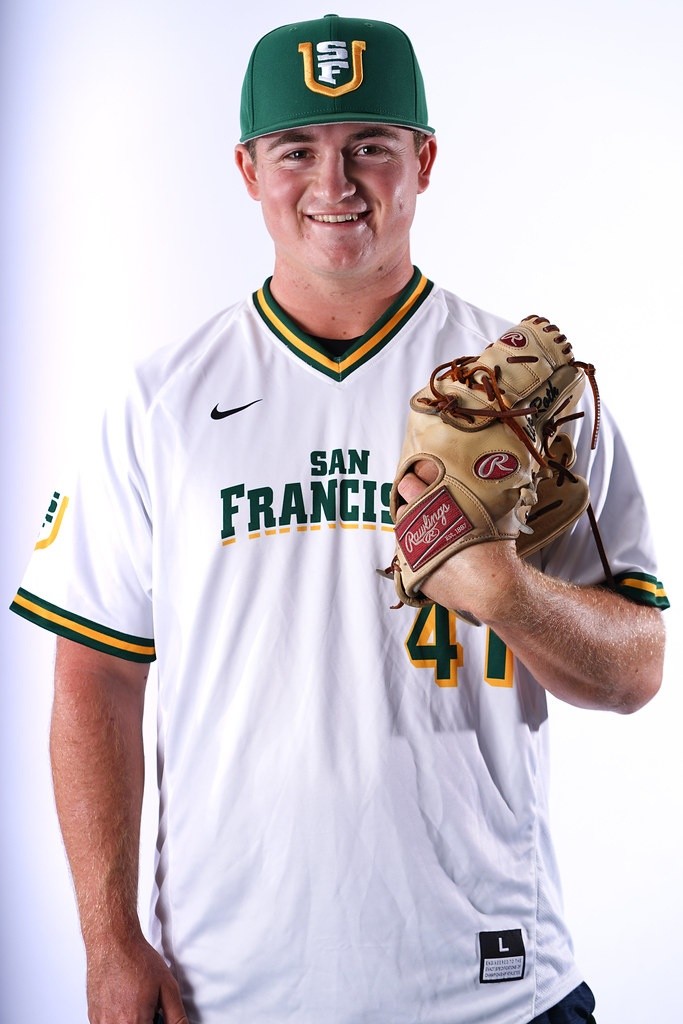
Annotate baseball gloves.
[387,311,604,610]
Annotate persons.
[7,12,672,1024]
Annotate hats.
[236,15,435,140]
